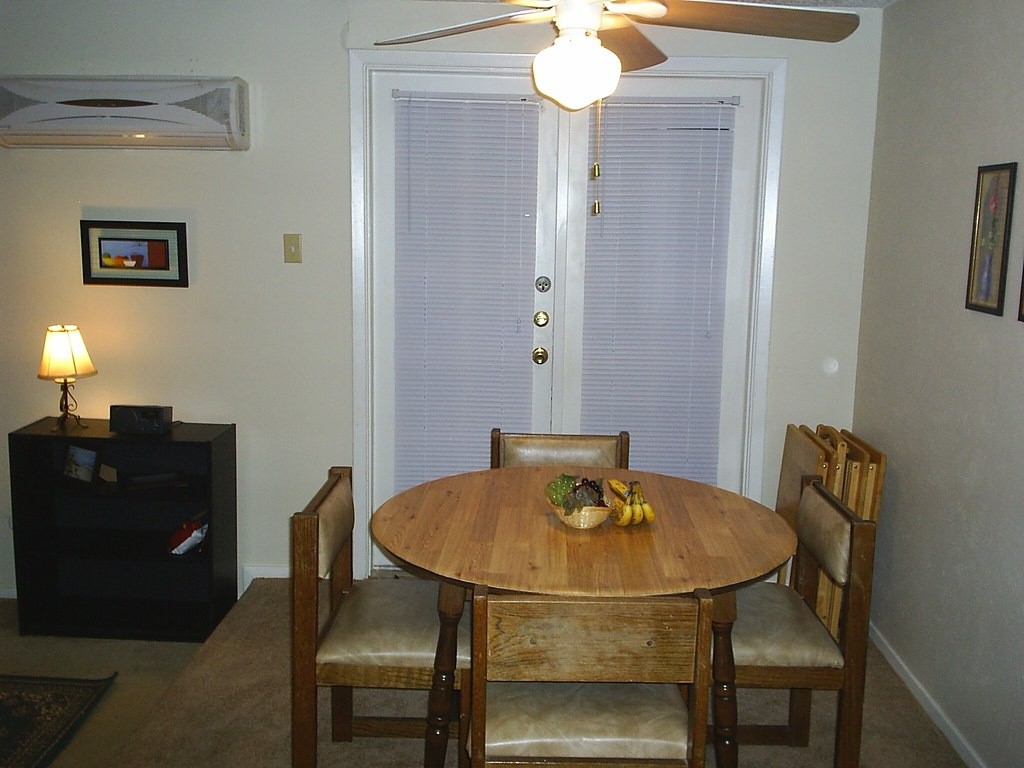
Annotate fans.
[373,0,860,73]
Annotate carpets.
[108,579,967,768]
[0,670,119,768]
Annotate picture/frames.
[964,161,1018,316]
[79,219,190,288]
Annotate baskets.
[546,497,613,529]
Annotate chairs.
[491,428,630,470]
[290,467,473,768]
[465,584,714,768]
[706,473,878,768]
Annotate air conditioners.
[0,73,250,150]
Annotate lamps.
[532,0,622,111]
[37,324,98,433]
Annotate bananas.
[609,478,655,527]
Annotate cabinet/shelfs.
[8,415,235,641]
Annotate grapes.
[547,475,604,504]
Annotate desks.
[367,463,798,768]
